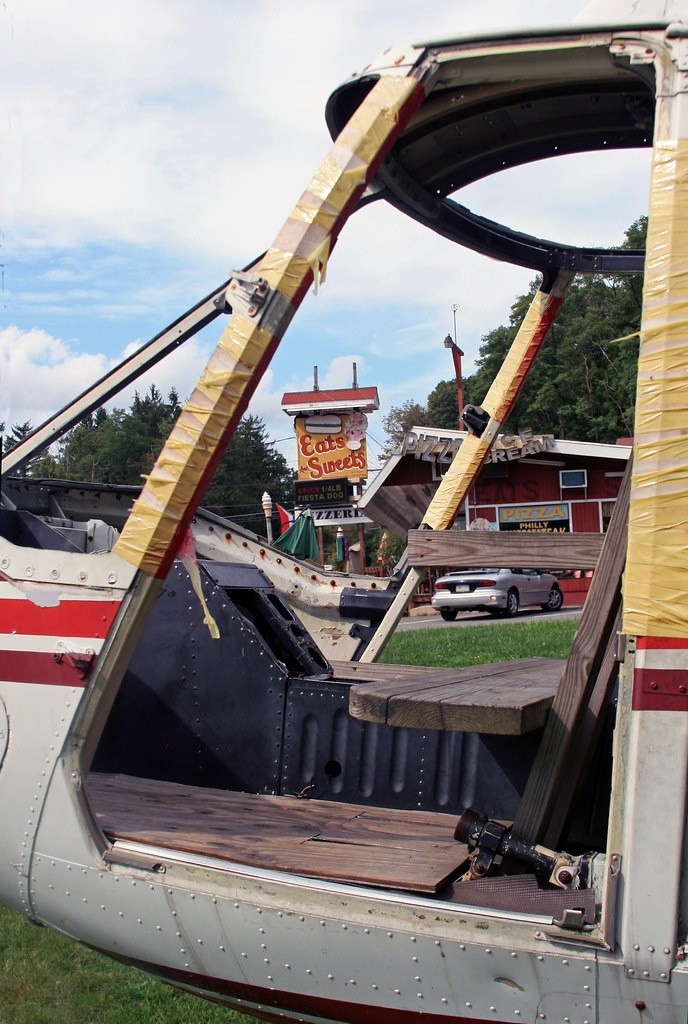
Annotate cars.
[431,568,563,620]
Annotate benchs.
[347,446,635,868]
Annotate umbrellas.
[270,504,320,561]
[336,526,350,562]
